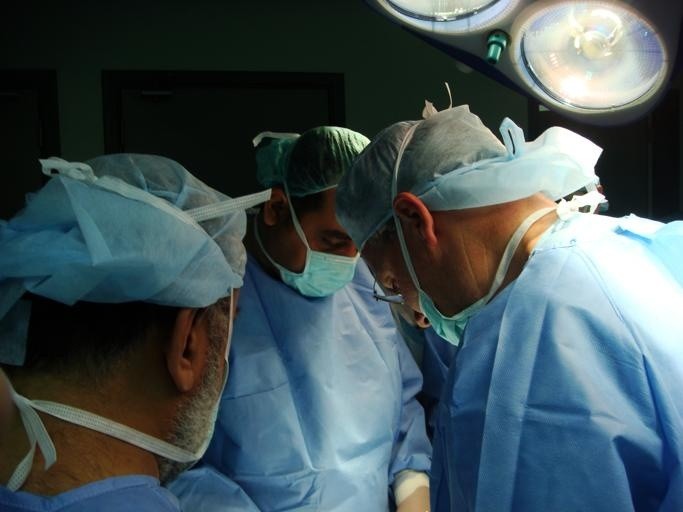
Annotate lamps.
[373,0,676,126]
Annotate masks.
[393,219,492,348]
[260,213,359,299]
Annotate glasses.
[371,278,407,306]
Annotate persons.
[0,152,248,511]
[187,124,434,510]
[334,103,682,510]
